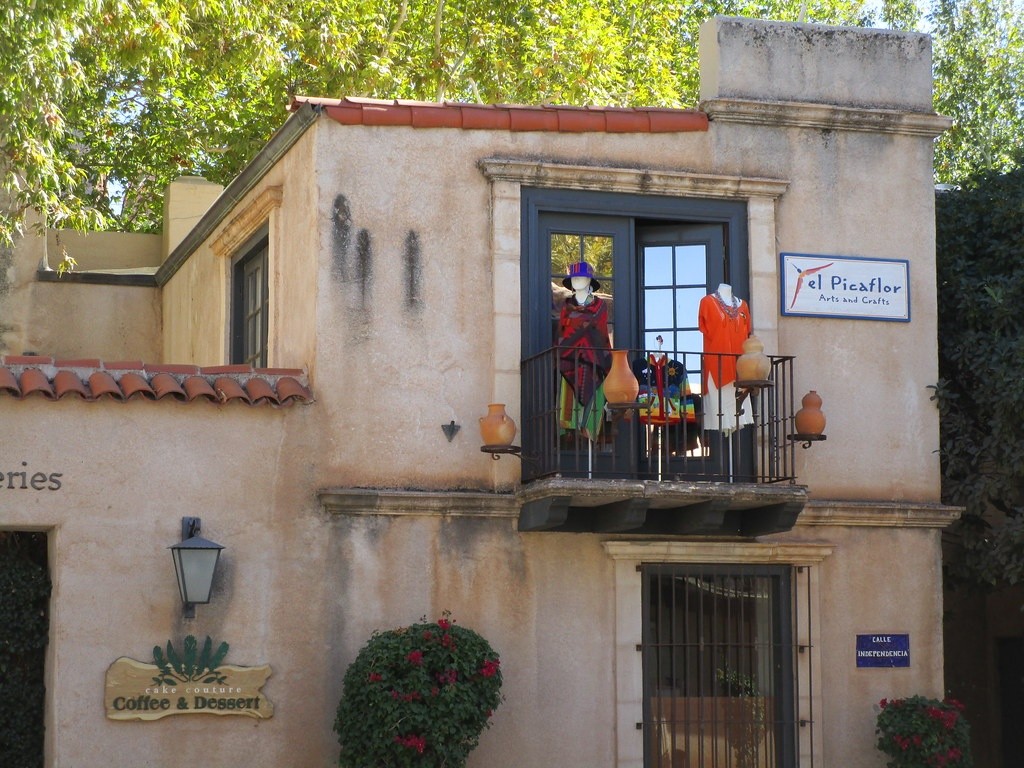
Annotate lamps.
[166,517,226,618]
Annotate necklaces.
[714,290,738,316]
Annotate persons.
[699,283,754,434]
[557,262,610,439]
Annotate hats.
[562,262,601,292]
